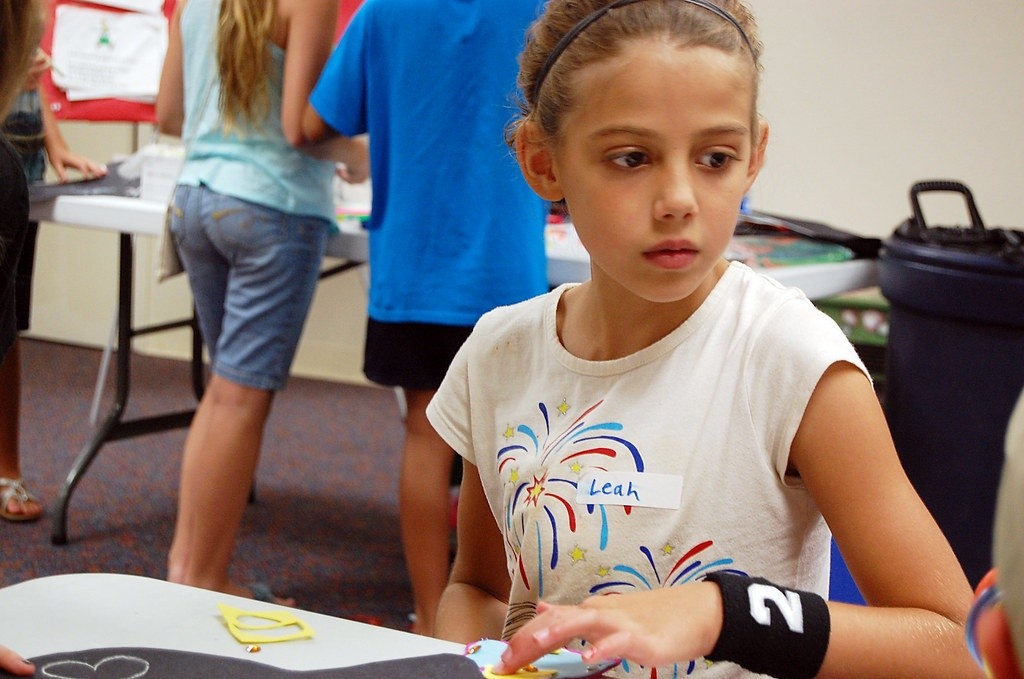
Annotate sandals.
[250,582,298,608]
[0,477,40,521]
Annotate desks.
[0,572,617,679]
[25,159,879,548]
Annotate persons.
[151,2,369,604]
[425,0,983,679]
[968,386,1024,679]
[302,1,565,639]
[0,2,105,524]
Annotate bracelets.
[965,577,1004,677]
